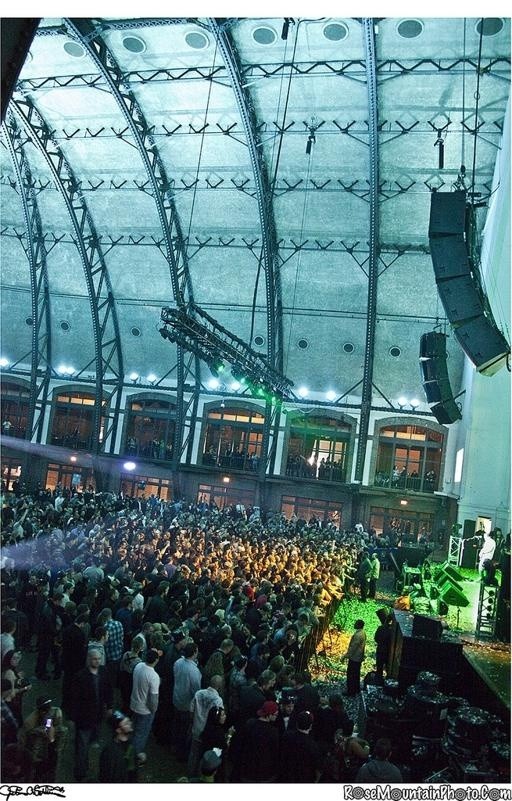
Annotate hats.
[257,700,279,716]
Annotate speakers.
[429,398,463,425]
[453,314,511,379]
[412,613,444,639]
[462,537,478,569]
[419,358,449,380]
[427,235,472,283]
[419,332,447,360]
[432,560,470,607]
[435,274,484,325]
[422,379,453,404]
[429,192,468,238]
[463,520,475,538]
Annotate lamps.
[157,42,303,409]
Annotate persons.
[1,442,510,783]
